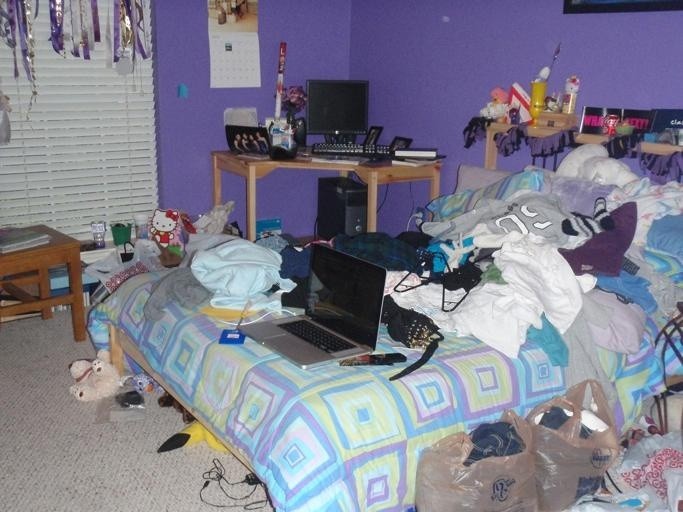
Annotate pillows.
[427,170,543,221]
[645,214,683,269]
[525,165,617,218]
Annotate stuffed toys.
[65,347,120,400]
[120,372,163,395]
[602,115,620,135]
[489,88,508,105]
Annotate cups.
[89,220,106,249]
[133,215,148,241]
[109,223,131,246]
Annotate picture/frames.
[563,0,683,14]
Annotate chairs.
[0,225,86,342]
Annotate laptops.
[236,242,388,370]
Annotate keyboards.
[312,143,392,156]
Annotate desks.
[212,149,444,242]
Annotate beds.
[107,119,683,511]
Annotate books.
[404,155,445,163]
[393,148,438,158]
[0,238,50,253]
[0,227,49,246]
[389,160,424,168]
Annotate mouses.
[366,155,394,168]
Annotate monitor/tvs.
[306,79,370,143]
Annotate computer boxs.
[316,176,367,243]
